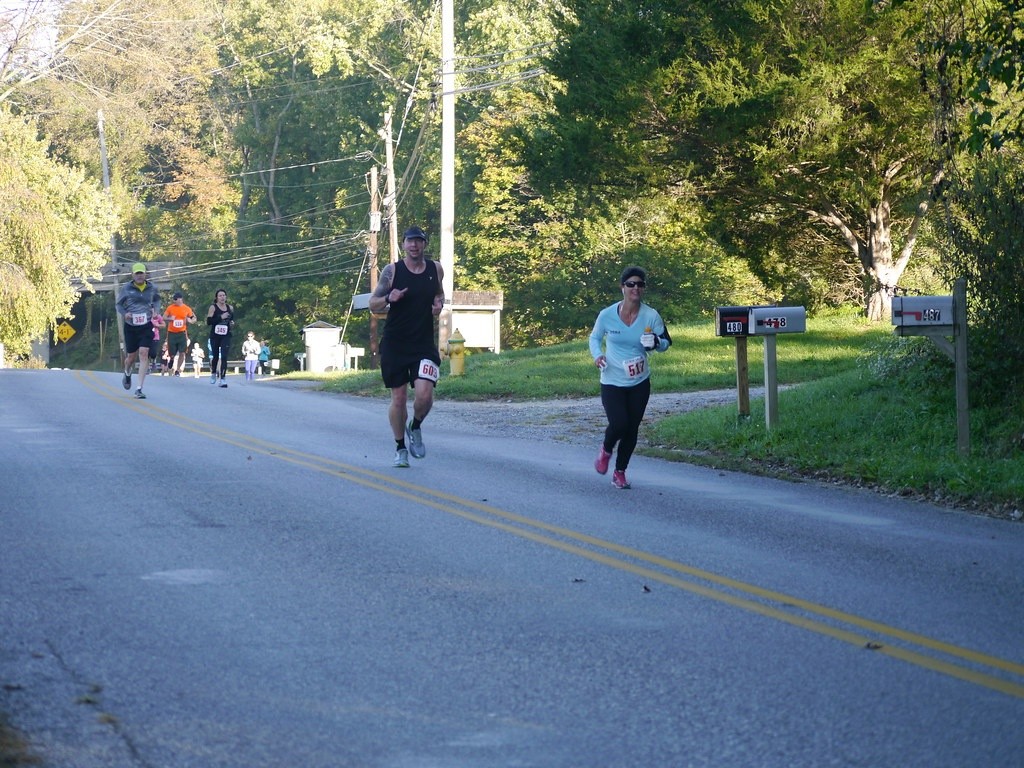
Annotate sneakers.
[610,469,631,489]
[595,443,613,474]
[123,370,131,390]
[392,449,409,467]
[135,389,146,398]
[406,419,425,458]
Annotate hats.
[132,262,146,273]
[403,228,427,242]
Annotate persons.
[162,292,197,377]
[208,338,221,379]
[242,331,261,381]
[207,288,235,388]
[590,267,671,489]
[191,343,205,379]
[370,227,445,467]
[115,262,161,398]
[146,304,190,376]
[258,342,270,374]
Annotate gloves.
[595,355,608,372]
[640,332,661,350]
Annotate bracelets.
[385,294,390,304]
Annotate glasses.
[622,281,646,288]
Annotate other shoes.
[218,379,227,388]
[210,373,216,384]
[175,371,180,377]
[168,359,173,369]
